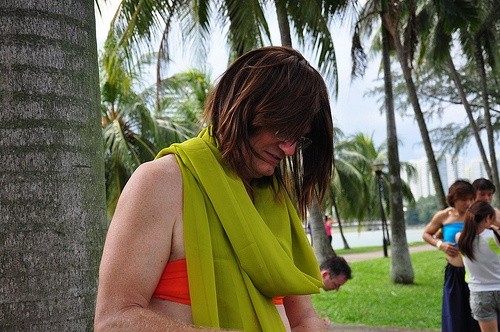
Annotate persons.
[322,215,335,247]
[318,256,351,292]
[422,178,500,332]
[94,46,335,332]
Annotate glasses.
[271,127,312,150]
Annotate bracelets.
[437,240,442,250]
